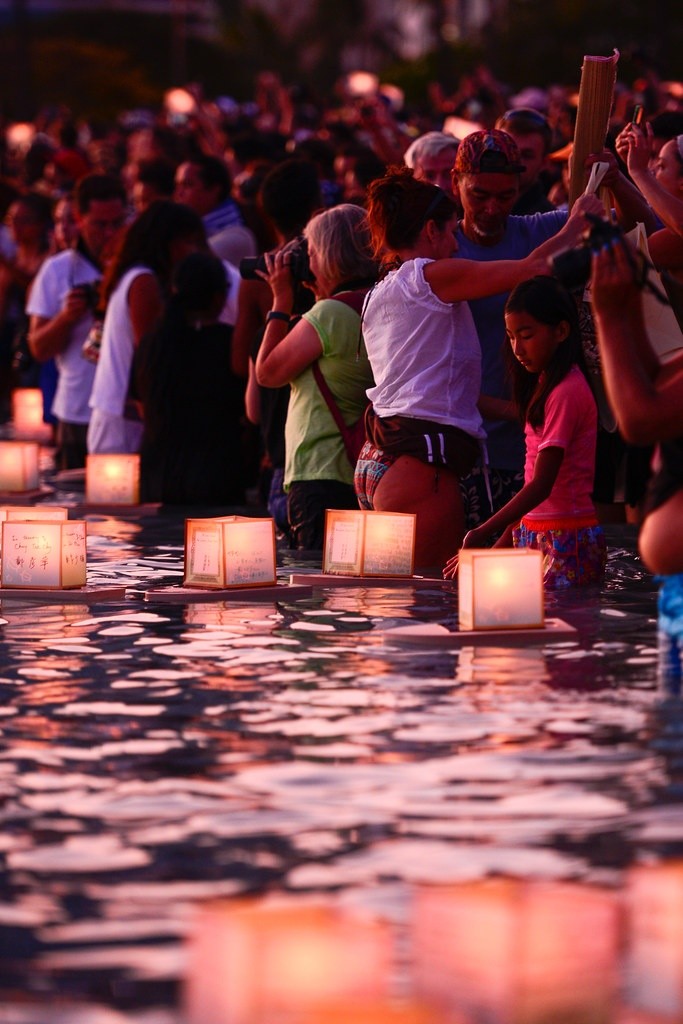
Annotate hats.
[454,129,527,174]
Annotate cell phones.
[630,105,645,132]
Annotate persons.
[0,0,683,580]
[440,272,611,603]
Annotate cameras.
[240,252,316,283]
[72,277,101,309]
[575,221,649,293]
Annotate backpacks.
[312,290,375,470]
